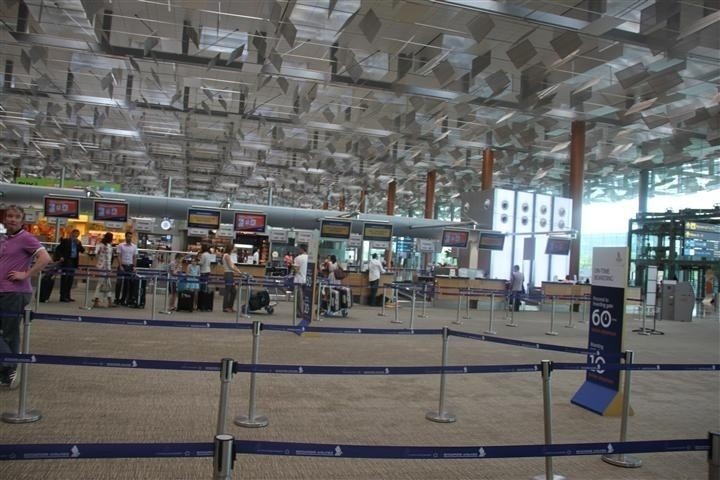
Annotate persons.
[94,233,116,308]
[166,245,339,319]
[368,254,386,306]
[56,229,84,302]
[115,232,138,306]
[509,265,524,311]
[0,205,51,385]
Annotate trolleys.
[318,284,354,318]
[239,272,278,317]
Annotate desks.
[541,281,593,305]
[434,274,508,311]
[318,271,394,305]
[183,260,266,297]
[78,253,121,269]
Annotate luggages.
[327,286,352,312]
[126,279,147,309]
[176,290,195,312]
[196,291,213,312]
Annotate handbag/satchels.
[335,267,345,280]
[249,291,270,311]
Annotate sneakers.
[0,363,17,390]
[93,299,126,308]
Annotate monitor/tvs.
[363,222,394,242]
[441,229,470,249]
[320,218,352,239]
[43,196,80,220]
[479,232,506,251]
[233,211,267,233]
[93,200,129,223]
[545,238,570,255]
[187,207,221,229]
